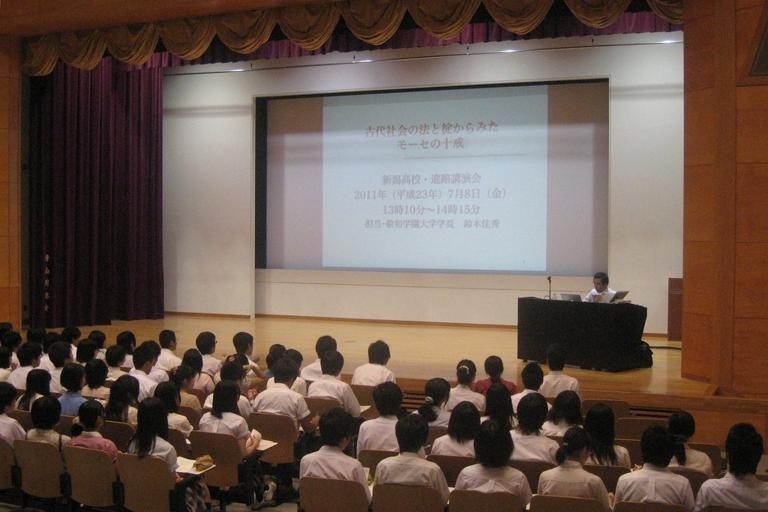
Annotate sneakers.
[263,480,277,501]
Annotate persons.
[410,350,582,479]
[582,272,617,303]
[456,420,534,504]
[66,399,120,505]
[583,405,712,474]
[128,396,211,512]
[695,423,768,511]
[373,413,452,507]
[613,423,694,512]
[300,407,373,507]
[1,382,27,486]
[199,379,278,512]
[1,322,398,456]
[537,427,609,512]
[25,396,72,496]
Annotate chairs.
[702,503,766,511]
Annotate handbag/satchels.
[641,340,653,368]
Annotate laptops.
[560,293,582,302]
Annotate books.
[592,291,631,304]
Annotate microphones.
[547,275,552,301]
